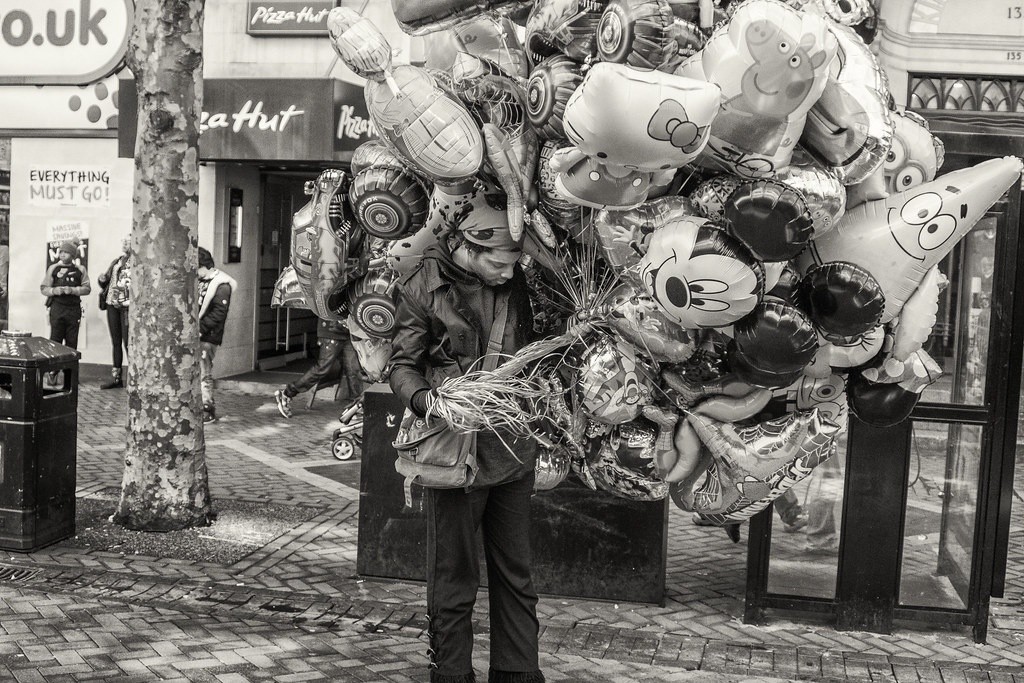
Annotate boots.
[100,368,123,389]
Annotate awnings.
[119,78,379,160]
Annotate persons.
[197,246,235,423]
[41,237,91,349]
[274,318,363,418]
[0,245,8,297]
[388,195,546,683]
[98,235,131,389]
[773,452,842,555]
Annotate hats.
[60,237,79,259]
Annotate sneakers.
[202,397,215,424]
[275,389,293,418]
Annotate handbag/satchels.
[394,406,478,510]
[99,289,107,310]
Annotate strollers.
[331,365,390,462]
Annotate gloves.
[417,386,446,418]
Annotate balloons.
[268,0,1024,544]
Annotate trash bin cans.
[0,329,82,554]
